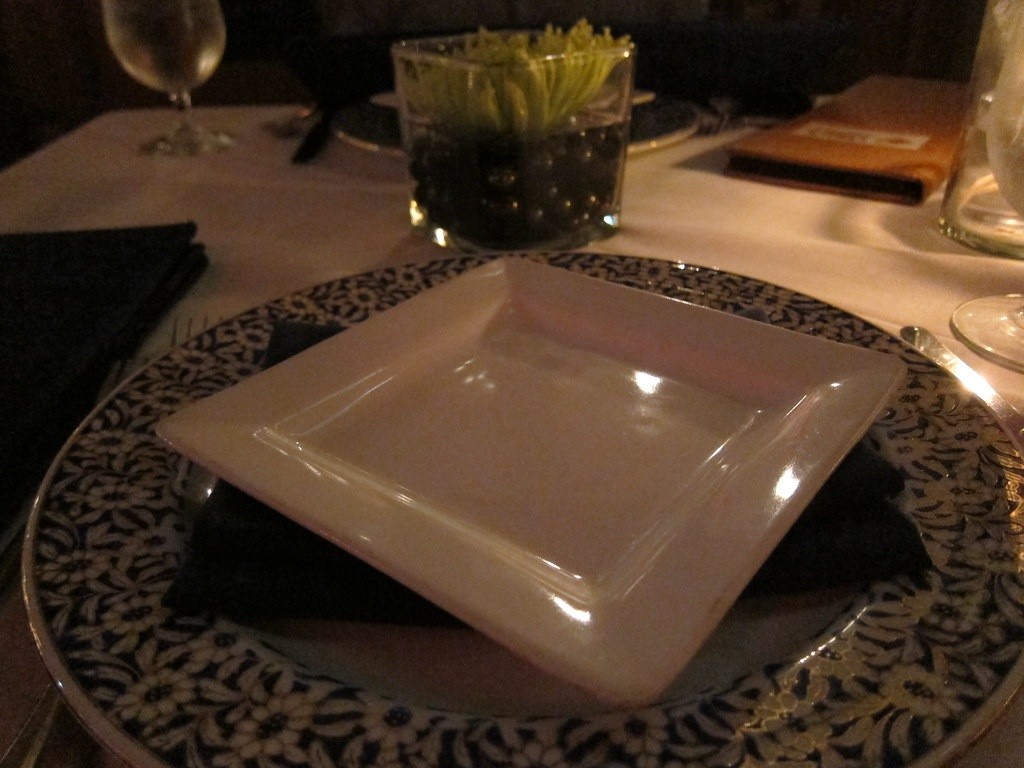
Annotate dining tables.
[0,108,1024,766]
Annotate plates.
[331,91,703,162]
[154,251,916,712]
[20,245,1024,767]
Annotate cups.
[937,0,1024,262]
[396,32,634,257]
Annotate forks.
[170,297,234,352]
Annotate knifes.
[287,65,369,164]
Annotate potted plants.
[393,18,633,254]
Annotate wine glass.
[99,0,235,154]
[948,14,1023,371]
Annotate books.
[724,75,978,206]
